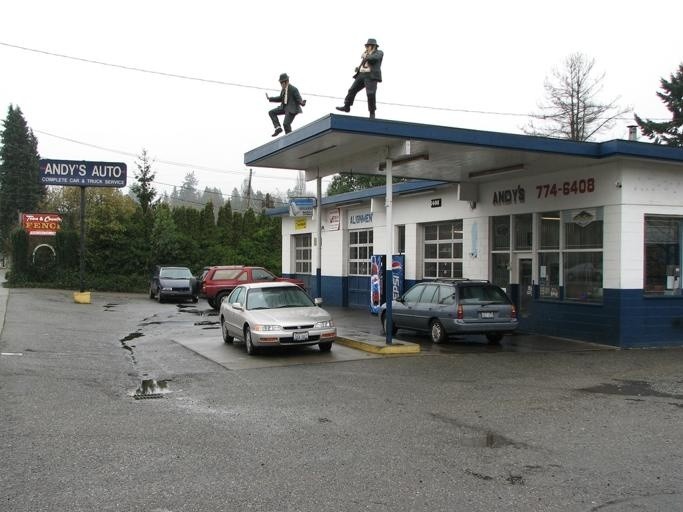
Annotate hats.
[365,39,379,48]
[279,73,289,83]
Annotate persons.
[335,38,384,122]
[263,72,306,138]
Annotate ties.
[363,53,369,68]
[281,90,285,110]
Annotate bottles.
[371,257,379,306]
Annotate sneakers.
[336,107,350,112]
[272,128,282,136]
[370,110,375,119]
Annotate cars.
[148,263,339,355]
[378,277,520,345]
[563,261,602,281]
